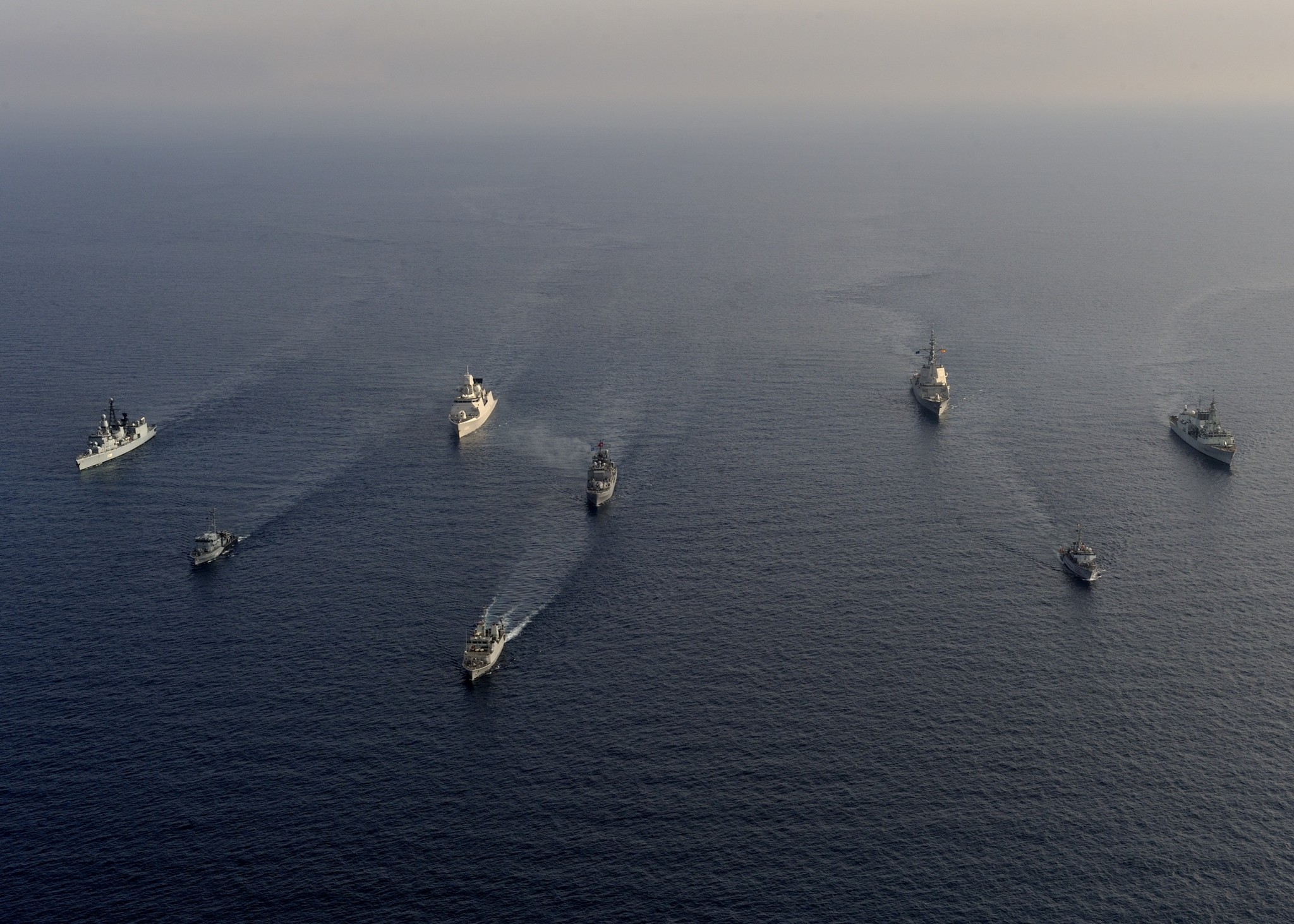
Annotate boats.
[462,618,506,681]
[584,438,618,505]
[189,507,240,565]
[1060,521,1100,580]
[447,365,498,438]
[908,328,952,418]
[1167,389,1237,465]
[75,397,159,472]
